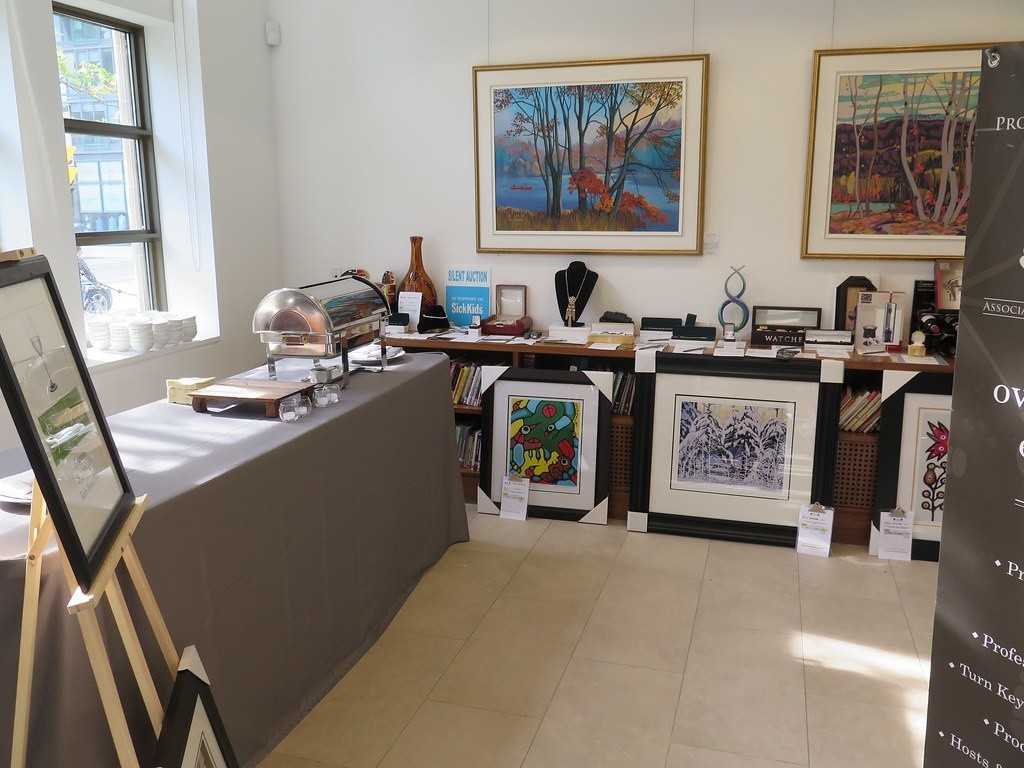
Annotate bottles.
[920,311,960,336]
[396,236,437,310]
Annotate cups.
[313,383,340,409]
[279,395,311,424]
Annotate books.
[597,364,635,416]
[838,385,884,434]
[444,353,504,472]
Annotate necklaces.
[563,267,588,328]
[423,314,449,321]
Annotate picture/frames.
[472,53,710,256]
[476,366,613,525]
[867,368,955,563]
[801,42,1011,260]
[146,645,240,768]
[0,255,134,593]
[627,351,844,552]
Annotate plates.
[0,473,32,505]
[350,348,405,364]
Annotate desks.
[0,351,468,768]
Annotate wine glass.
[18,314,57,393]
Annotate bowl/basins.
[85,309,198,355]
